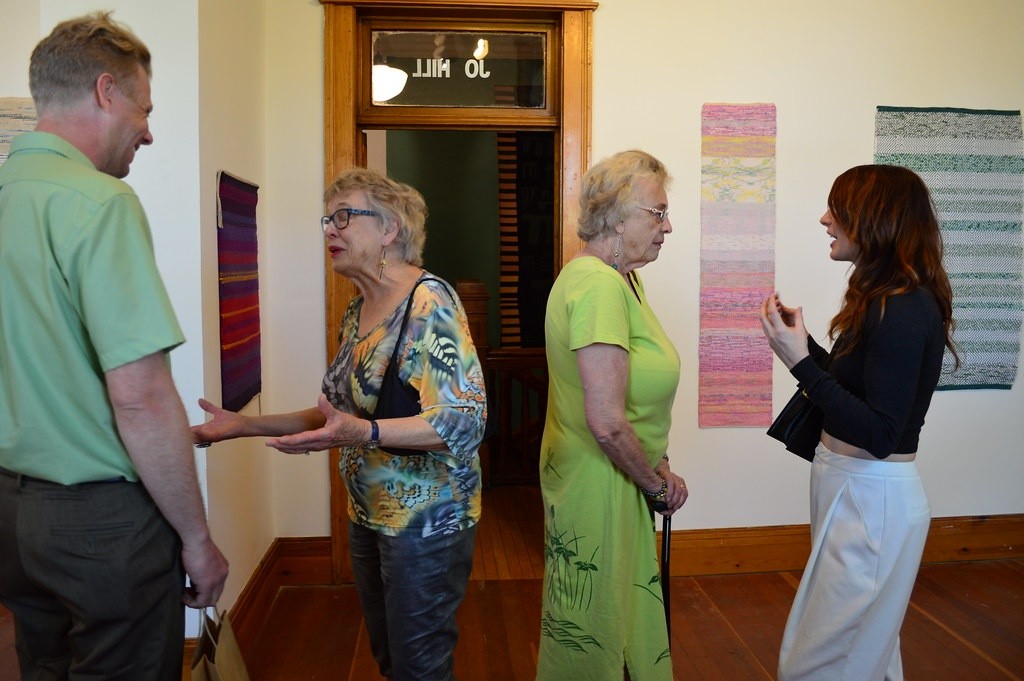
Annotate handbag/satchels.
[370,278,460,455]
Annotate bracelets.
[662,452,669,461]
[641,474,666,499]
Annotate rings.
[304,448,311,456]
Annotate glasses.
[632,202,671,221]
[321,209,387,227]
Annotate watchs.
[363,419,382,450]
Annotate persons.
[535,149,688,681]
[759,164,963,681]
[0,6,228,681]
[186,165,489,681]
